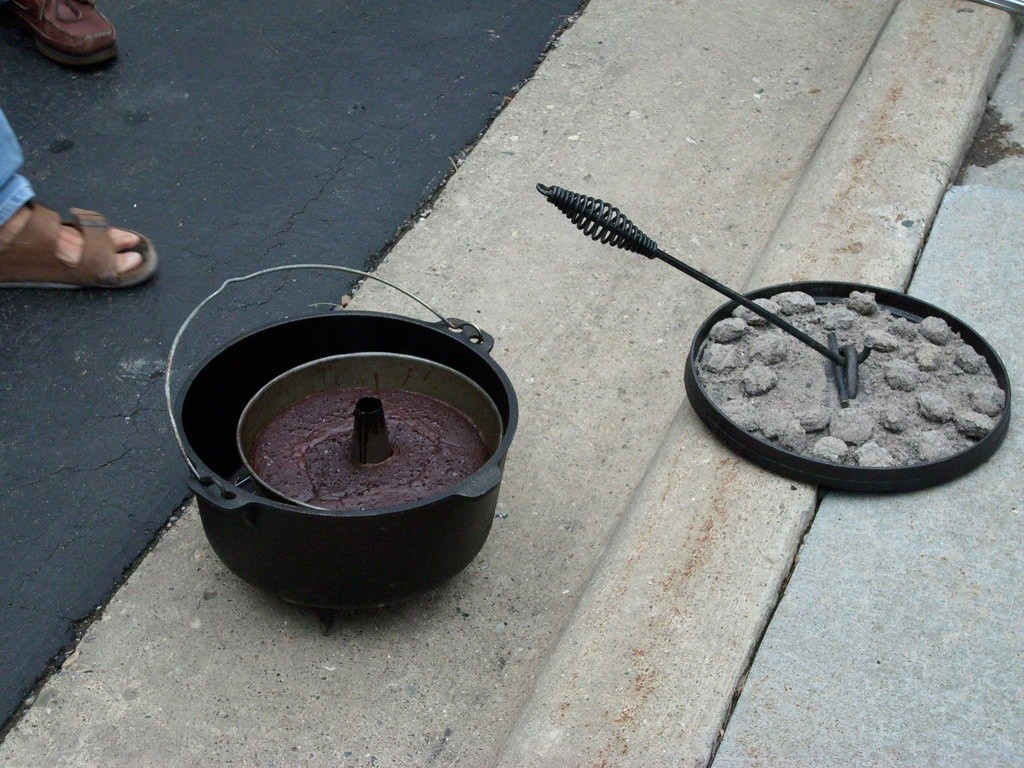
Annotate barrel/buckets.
[163,262,520,614]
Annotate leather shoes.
[0,0,118,66]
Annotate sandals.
[0,200,159,288]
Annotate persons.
[0,110,159,289]
[11,0,119,64]
